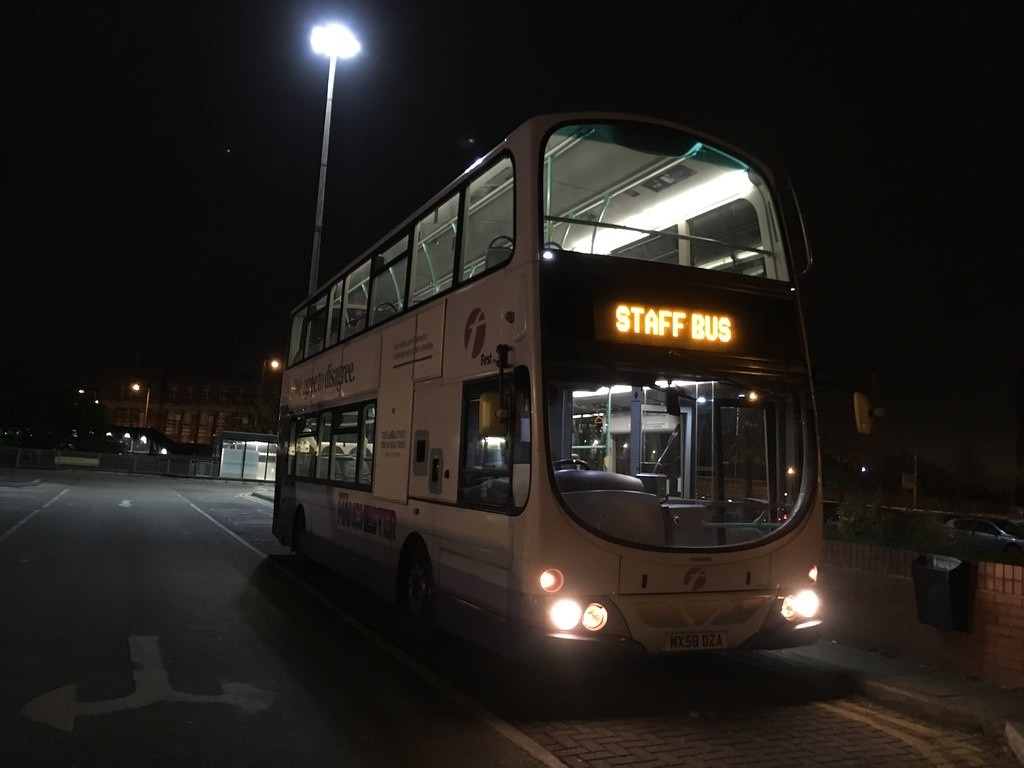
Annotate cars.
[945,518,1024,547]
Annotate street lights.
[133,384,149,427]
[254,359,278,433]
[308,23,360,297]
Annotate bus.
[271,111,823,666]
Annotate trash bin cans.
[910,553,972,633]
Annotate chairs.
[484,234,513,269]
[374,302,397,324]
[321,446,345,469]
[341,454,370,484]
[349,447,372,456]
[345,319,359,337]
[291,451,317,477]
[502,410,530,466]
[320,453,341,479]
